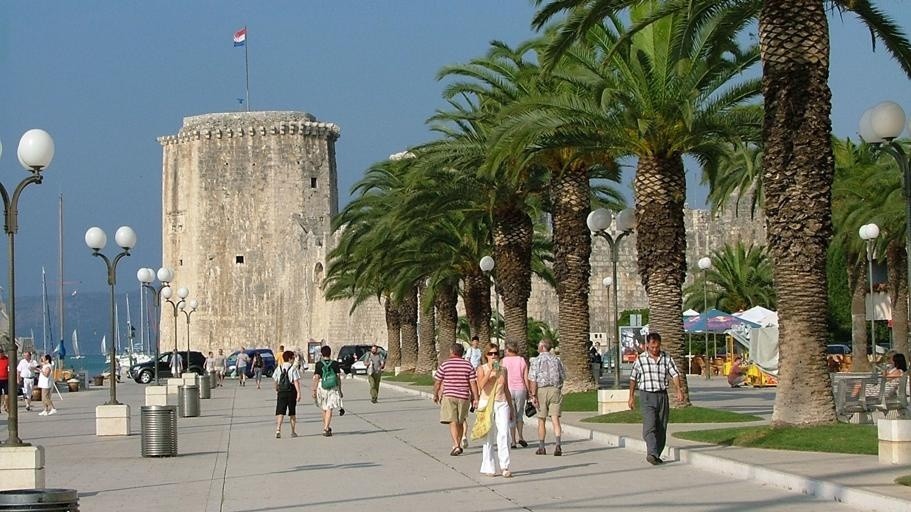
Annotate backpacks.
[321,359,337,389]
[256,355,262,367]
[279,365,293,392]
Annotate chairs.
[696,356,726,377]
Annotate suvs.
[130,351,210,384]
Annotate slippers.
[511,440,528,448]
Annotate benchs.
[833,376,909,424]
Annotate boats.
[14,333,58,370]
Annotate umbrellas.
[681,305,782,374]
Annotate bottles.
[494,360,501,371]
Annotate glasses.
[487,352,499,356]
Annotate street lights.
[603,277,615,374]
[0,129,57,490]
[137,265,175,406]
[160,286,190,394]
[480,256,500,358]
[177,299,200,386]
[699,257,713,380]
[81,224,138,437]
[587,207,638,414]
[858,100,911,487]
[860,223,881,373]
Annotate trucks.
[554,333,608,356]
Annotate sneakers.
[275,430,280,438]
[450,437,468,456]
[38,408,56,416]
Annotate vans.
[223,347,277,378]
[337,344,387,374]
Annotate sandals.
[323,427,332,436]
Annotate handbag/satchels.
[470,399,494,440]
[525,397,536,417]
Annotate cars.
[828,344,853,360]
[351,352,387,375]
[867,342,889,362]
[602,346,617,368]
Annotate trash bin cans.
[0,488,80,512]
[141,405,177,457]
[93,375,104,386]
[195,375,210,399]
[178,384,200,417]
[31,385,42,401]
[204,370,216,389]
[67,378,80,392]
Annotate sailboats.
[36,266,80,380]
[98,334,110,356]
[69,327,87,360]
[106,282,156,366]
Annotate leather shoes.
[554,445,561,456]
[536,448,546,455]
[646,455,663,465]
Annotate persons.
[30,354,58,416]
[0,348,11,412]
[272,351,301,438]
[169,348,184,377]
[203,351,217,376]
[275,346,284,367]
[465,336,482,369]
[249,352,264,389]
[848,352,907,402]
[363,345,384,403]
[727,357,749,388]
[498,342,529,448]
[476,343,514,478]
[433,343,478,455]
[235,346,251,387]
[312,345,343,437]
[16,350,42,411]
[212,348,227,387]
[527,337,566,455]
[628,332,686,466]
